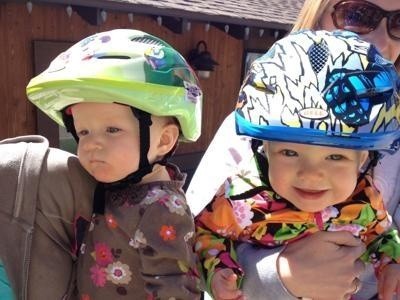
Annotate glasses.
[331,0,400,43]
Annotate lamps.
[187,38,220,79]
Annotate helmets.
[25,27,204,143]
[234,27,400,151]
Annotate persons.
[24,27,208,300]
[193,28,399,300]
[184,1,400,232]
